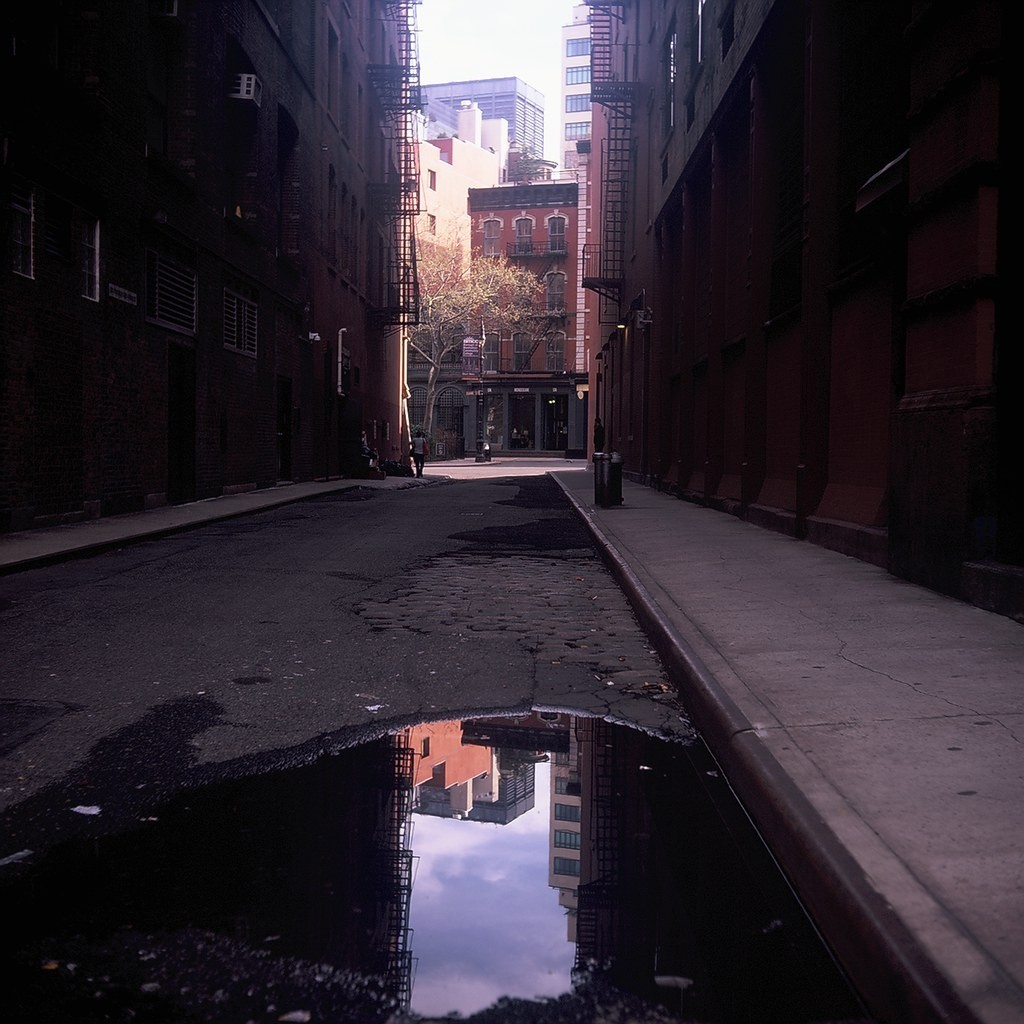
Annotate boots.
[416,469,424,477]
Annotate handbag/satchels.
[422,438,430,456]
[408,442,416,457]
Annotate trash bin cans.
[592,451,623,506]
[435,442,449,461]
[483,440,491,462]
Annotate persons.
[408,432,426,478]
[594,418,606,452]
